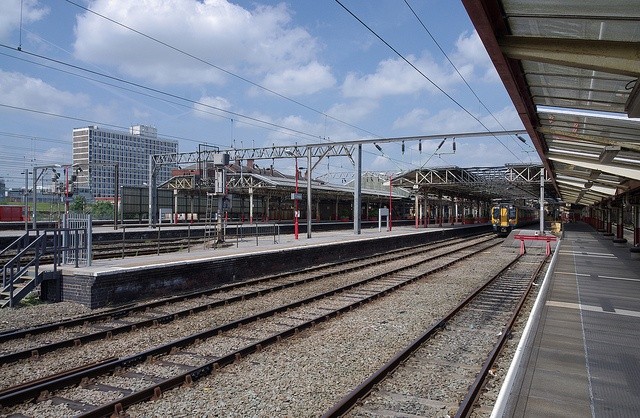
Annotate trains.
[491,202,548,237]
